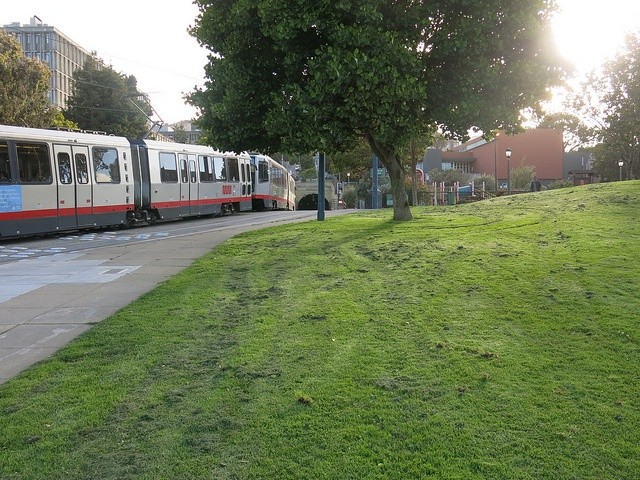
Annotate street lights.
[618,159,623,181]
[506,148,512,195]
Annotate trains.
[0,124,297,241]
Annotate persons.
[531,176,542,192]
